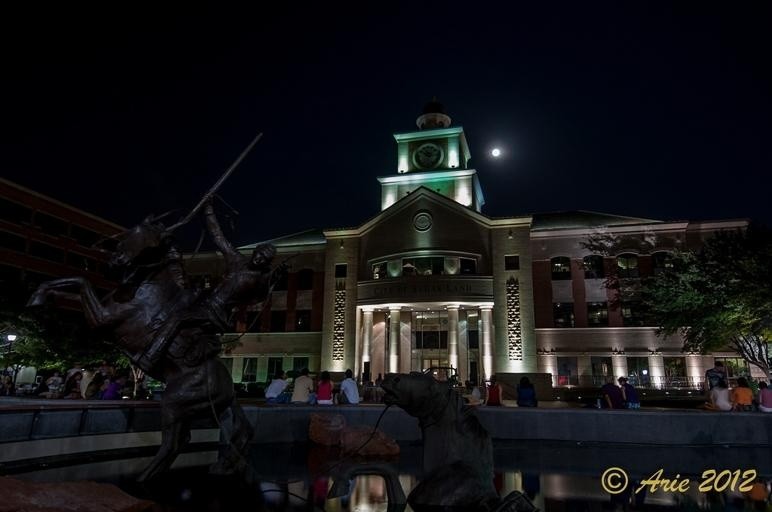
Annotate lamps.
[5,334,17,370]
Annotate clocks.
[415,145,441,169]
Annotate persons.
[710,380,733,412]
[265,370,289,404]
[291,368,315,406]
[337,369,360,406]
[705,361,728,393]
[617,377,640,409]
[483,374,504,408]
[138,194,290,372]
[463,380,481,405]
[599,382,624,409]
[0,366,146,404]
[758,381,772,413]
[362,382,374,402]
[516,376,538,408]
[316,370,334,405]
[375,372,384,402]
[732,376,755,412]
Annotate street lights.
[4,333,17,377]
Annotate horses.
[27,211,258,483]
[377,371,537,510]
